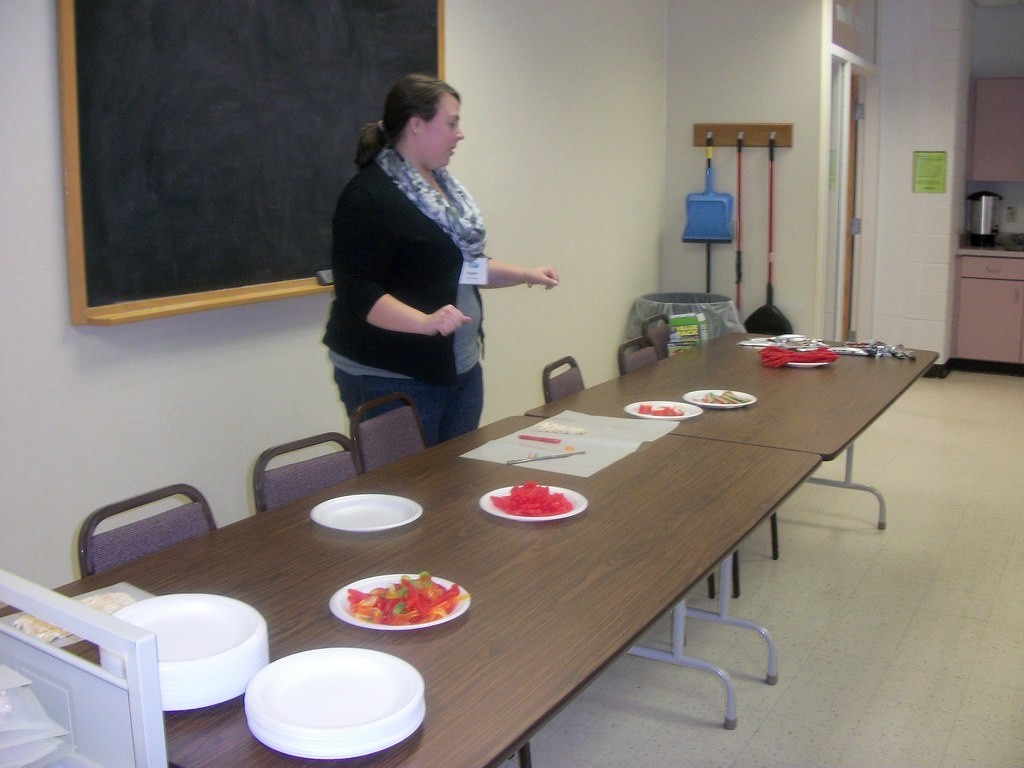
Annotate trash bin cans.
[639,291,732,357]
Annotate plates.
[98,593,270,710]
[479,485,588,523]
[329,573,471,630]
[682,390,757,409]
[244,647,426,760]
[309,494,423,531]
[624,401,704,421]
[784,361,830,368]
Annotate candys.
[703,391,746,404]
[489,480,574,516]
[639,403,685,416]
[346,571,471,625]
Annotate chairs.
[543,314,779,599]
[348,393,427,477]
[79,484,219,580]
[253,432,533,767]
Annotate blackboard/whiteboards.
[55,0,447,327]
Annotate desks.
[523,333,940,686]
[0,413,822,768]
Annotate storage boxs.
[668,313,707,356]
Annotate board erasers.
[316,270,336,286]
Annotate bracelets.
[527,267,532,288]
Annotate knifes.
[508,451,586,465]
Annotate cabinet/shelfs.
[951,255,1024,365]
[970,78,1024,182]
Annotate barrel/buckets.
[966,191,1003,247]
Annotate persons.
[324,75,560,448]
